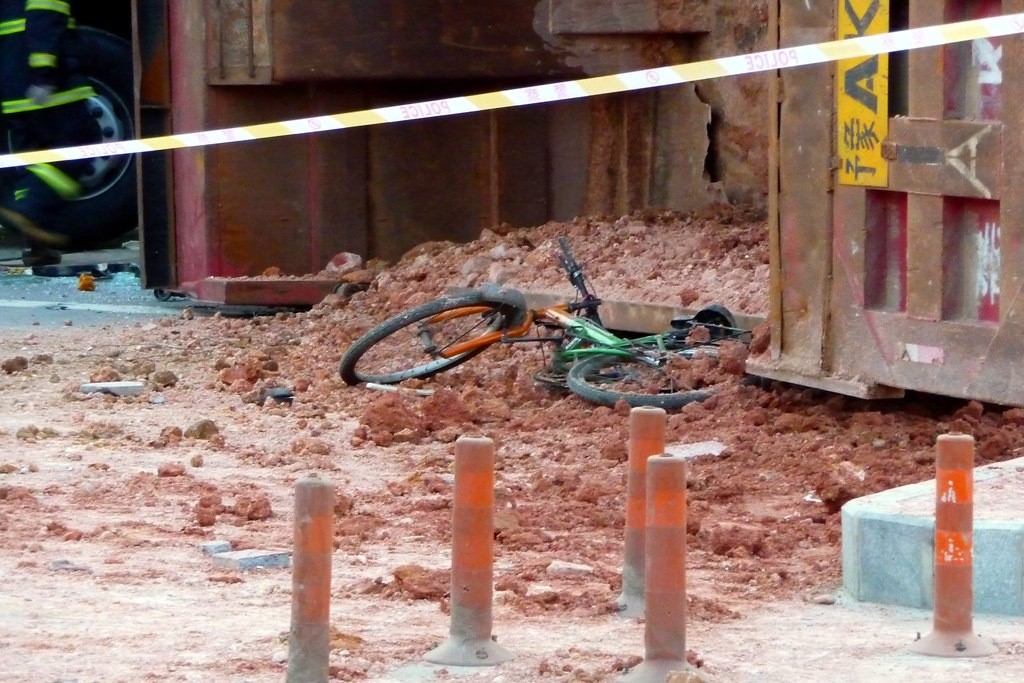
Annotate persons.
[0,0,104,266]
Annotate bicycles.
[336,237,760,407]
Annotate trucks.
[130,1,1022,414]
